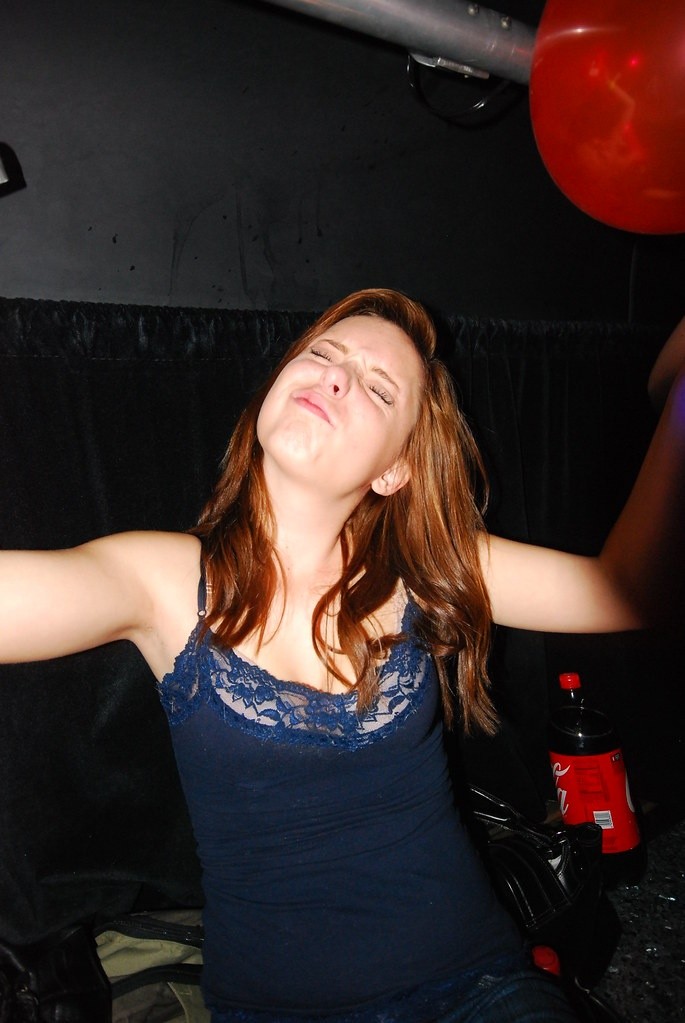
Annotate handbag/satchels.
[451,776,624,991]
[0,814,203,1023]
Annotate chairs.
[0,785,626,1023]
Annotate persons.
[0,288,685,1023]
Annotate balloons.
[529,0,685,236]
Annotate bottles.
[551,673,645,891]
